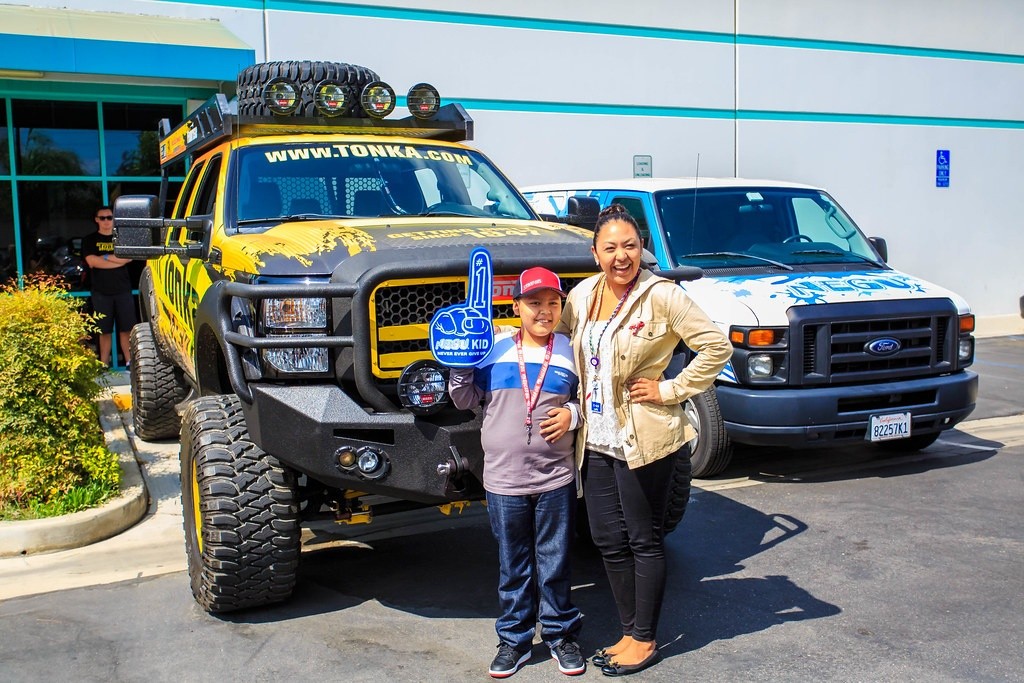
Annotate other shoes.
[591,647,617,667]
[96,368,108,375]
[602,640,662,676]
[125,365,131,374]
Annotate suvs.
[112,57,704,615]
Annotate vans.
[484,177,980,479]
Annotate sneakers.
[488,641,532,678]
[550,637,586,675]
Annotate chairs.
[288,198,322,216]
[252,182,282,217]
[353,191,390,216]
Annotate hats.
[512,266,566,300]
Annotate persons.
[553,203,734,677]
[448,266,584,679]
[81,206,136,374]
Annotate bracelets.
[104,253,108,260]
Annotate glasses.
[97,215,113,221]
